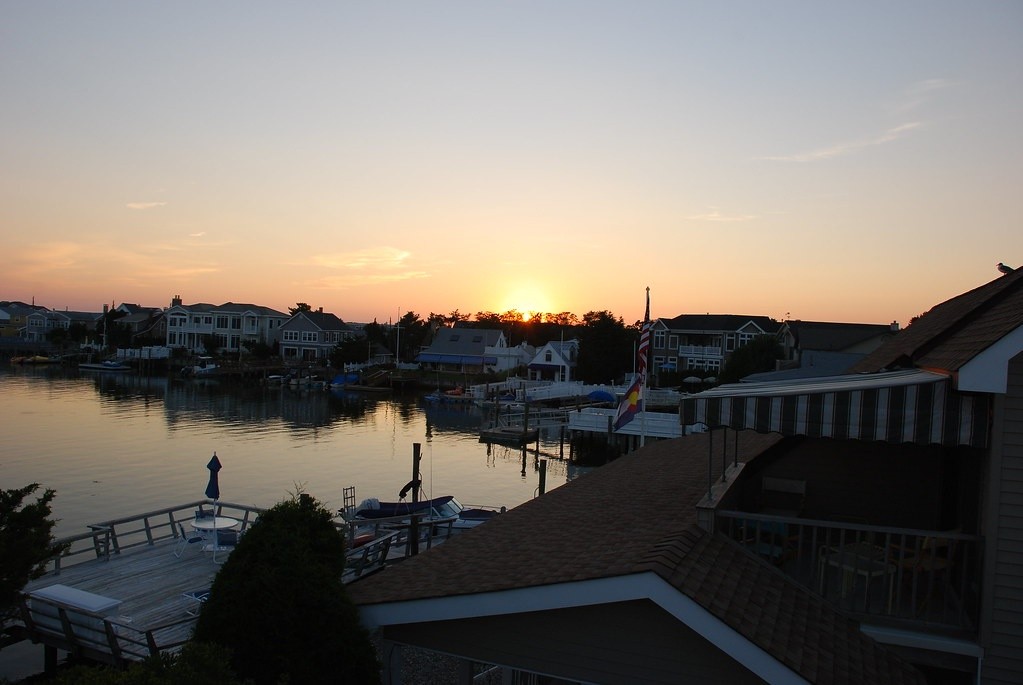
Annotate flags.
[610,375,644,432]
[637,297,650,370]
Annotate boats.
[78,350,368,397]
[338,494,505,537]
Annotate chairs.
[180,588,212,617]
[171,521,206,561]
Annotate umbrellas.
[204,450,223,519]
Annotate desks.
[190,516,240,562]
